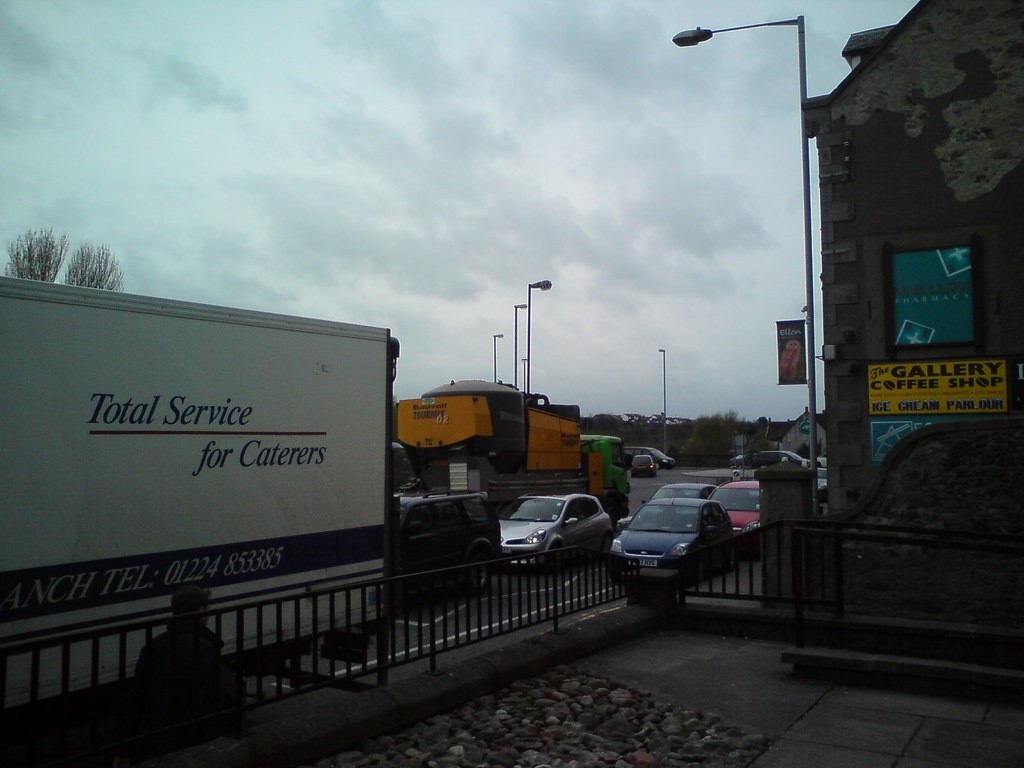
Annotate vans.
[623,447,676,470]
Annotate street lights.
[659,349,668,453]
[492,334,504,383]
[673,14,817,513]
[514,303,528,388]
[526,280,553,393]
[520,358,528,395]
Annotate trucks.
[394,379,632,527]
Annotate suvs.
[389,491,501,604]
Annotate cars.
[704,480,762,554]
[756,451,810,469]
[631,454,657,478]
[494,493,612,574]
[818,453,827,468]
[606,499,734,584]
[641,483,716,504]
